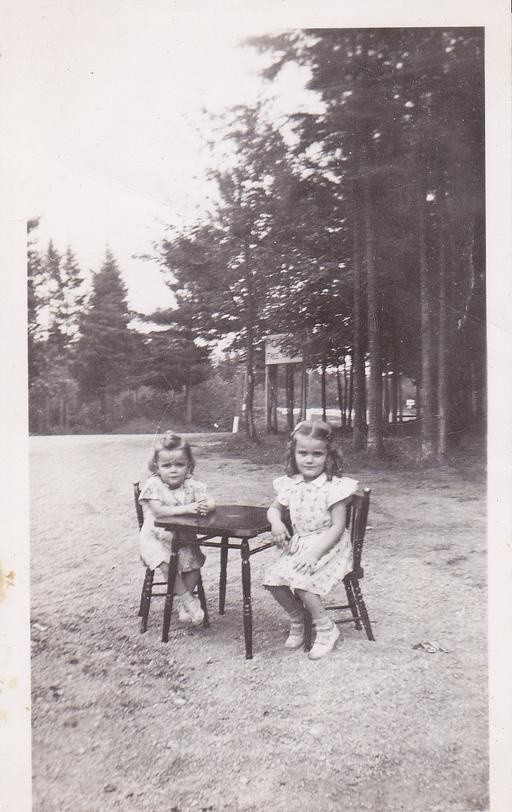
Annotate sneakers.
[285,623,305,649]
[187,598,204,626]
[308,621,340,660]
[176,600,192,624]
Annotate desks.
[154,505,294,660]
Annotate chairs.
[288,488,375,653]
[132,475,210,628]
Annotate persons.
[260,419,358,662]
[137,432,217,627]
